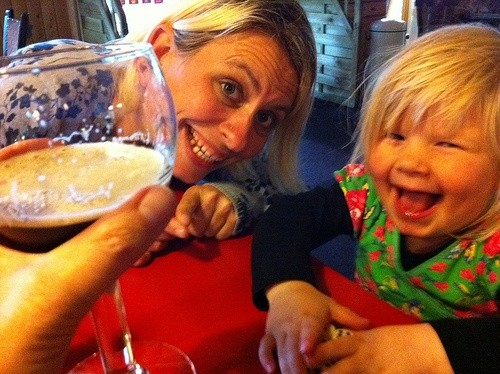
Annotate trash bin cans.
[367,18,407,76]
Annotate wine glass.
[0,42,197,374]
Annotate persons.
[250,23,500,374]
[0,0,317,266]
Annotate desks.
[67,234,420,374]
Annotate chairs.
[2,9,32,56]
